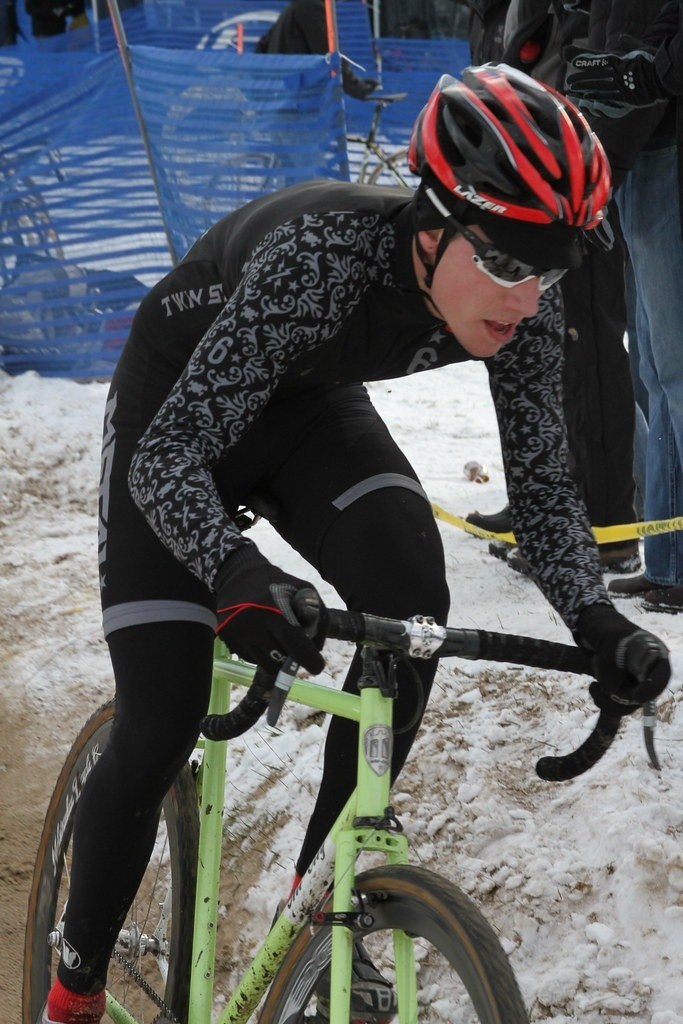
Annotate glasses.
[424,182,569,292]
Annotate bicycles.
[202,91,424,215]
[0,139,72,268]
[21,590,661,1024]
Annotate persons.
[464,0,683,613]
[34,61,673,1024]
[0,0,86,52]
[238,0,380,180]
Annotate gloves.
[572,605,672,717]
[215,544,327,677]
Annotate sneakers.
[466,506,514,541]
[313,938,398,1022]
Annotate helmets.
[409,61,615,272]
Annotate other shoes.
[645,588,683,607]
[488,538,642,575]
[610,573,676,594]
